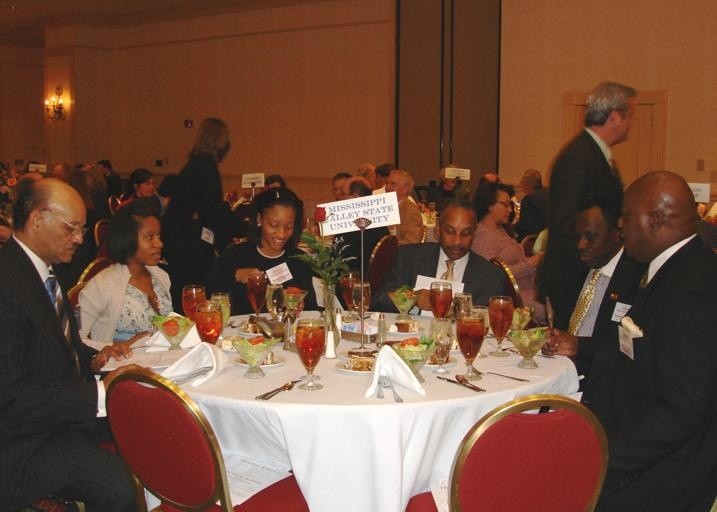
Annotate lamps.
[44,87,65,121]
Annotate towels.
[145,312,201,352]
[312,276,346,312]
[160,342,228,387]
[365,344,425,399]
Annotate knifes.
[546,295,555,349]
[436,374,482,392]
[255,377,302,399]
[508,348,555,360]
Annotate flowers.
[286,206,358,323]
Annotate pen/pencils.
[546,297,556,354]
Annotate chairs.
[366,235,398,291]
[407,393,610,512]
[511,200,520,224]
[102,363,310,512]
[93,219,110,247]
[490,256,524,309]
[108,196,121,216]
[76,257,112,284]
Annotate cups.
[182,285,204,320]
[247,273,267,316]
[353,281,370,318]
[195,303,223,346]
[489,296,514,357]
[386,290,421,322]
[283,288,309,311]
[429,323,453,364]
[153,317,193,351]
[210,292,230,326]
[296,319,325,391]
[232,340,271,379]
[473,303,489,336]
[510,330,544,369]
[457,312,484,381]
[429,281,453,318]
[454,292,473,320]
[392,342,430,383]
[340,270,361,320]
[266,284,286,316]
[512,312,532,331]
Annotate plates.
[235,354,288,375]
[335,358,377,377]
[386,328,420,338]
[234,328,264,338]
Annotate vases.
[321,284,342,359]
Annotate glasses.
[496,199,510,207]
[39,208,88,237]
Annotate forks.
[377,375,386,400]
[381,373,403,403]
[483,366,531,383]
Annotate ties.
[445,259,455,281]
[567,270,601,335]
[47,271,81,376]
[639,269,649,287]
[609,151,615,175]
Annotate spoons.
[265,381,295,400]
[455,374,488,390]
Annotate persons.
[542,170,717,512]
[426,164,471,210]
[203,187,318,318]
[82,164,107,210]
[384,170,423,247]
[536,82,637,308]
[513,169,549,242]
[179,118,227,225]
[265,175,286,190]
[330,176,389,272]
[480,173,500,184]
[16,173,47,196]
[374,200,507,315]
[0,179,138,512]
[52,161,72,184]
[700,200,716,252]
[470,182,547,326]
[115,168,165,217]
[553,196,644,337]
[76,194,173,353]
[156,175,224,315]
[332,163,419,207]
[96,160,123,196]
